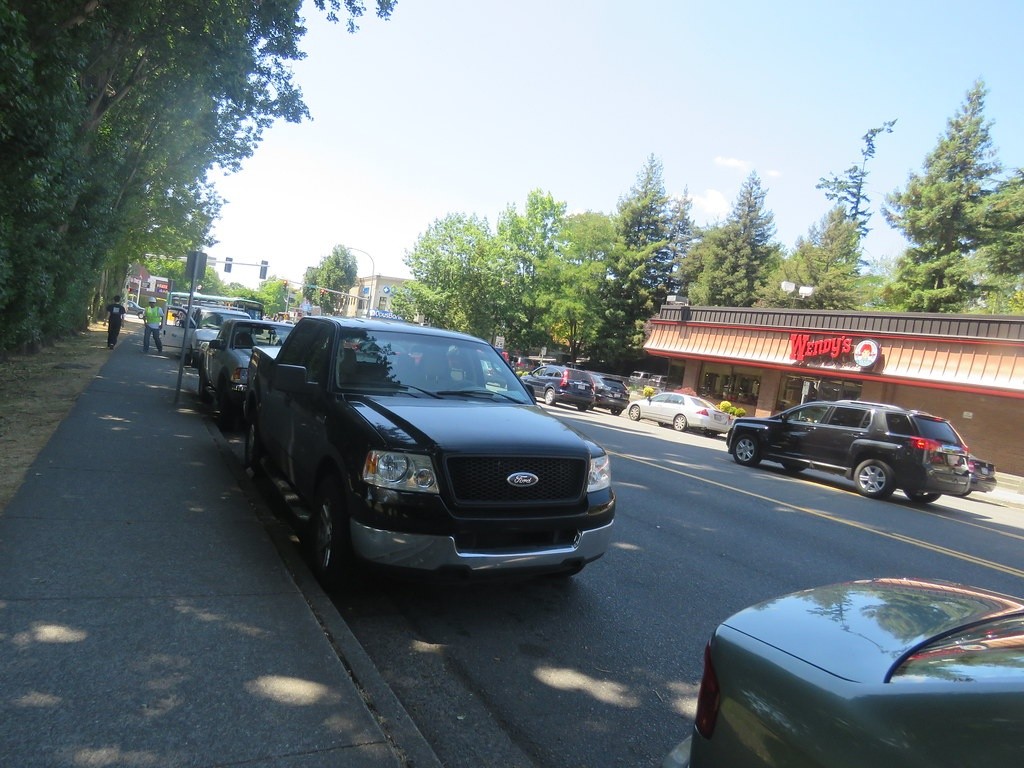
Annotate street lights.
[347,247,375,316]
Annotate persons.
[103,295,126,350]
[142,297,165,352]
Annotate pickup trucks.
[248,316,617,593]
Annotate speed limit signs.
[494,335,504,349]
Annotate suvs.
[724,399,971,506]
[127,300,147,320]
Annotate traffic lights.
[283,281,288,291]
[320,290,325,300]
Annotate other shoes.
[158,349,162,355]
[110,343,114,350]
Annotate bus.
[166,292,264,336]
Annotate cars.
[626,392,733,435]
[663,577,1024,768]
[954,455,999,497]
[588,371,630,416]
[480,360,508,388]
[520,365,597,412]
[163,304,250,368]
[198,320,298,426]
[171,305,202,327]
[281,320,293,325]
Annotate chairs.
[418,351,455,386]
[342,345,363,374]
[235,330,255,346]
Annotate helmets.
[148,296,156,303]
